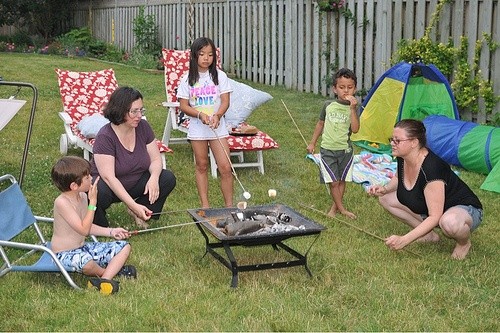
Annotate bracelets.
[88,205,96,210]
[198,111,201,119]
[213,114,221,120]
[110,229,113,235]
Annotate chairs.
[54,67,175,160]
[0,174,100,293]
[160,47,282,176]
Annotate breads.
[241,126,257,133]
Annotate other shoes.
[115,265,137,280]
[86,277,120,297]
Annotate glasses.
[388,137,416,145]
[128,107,146,116]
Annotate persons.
[306,68,360,219]
[369,119,482,259]
[174,38,233,208]
[51,157,136,294]
[90,87,176,228]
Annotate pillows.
[223,77,274,131]
[76,113,110,138]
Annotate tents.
[349,58,459,147]
